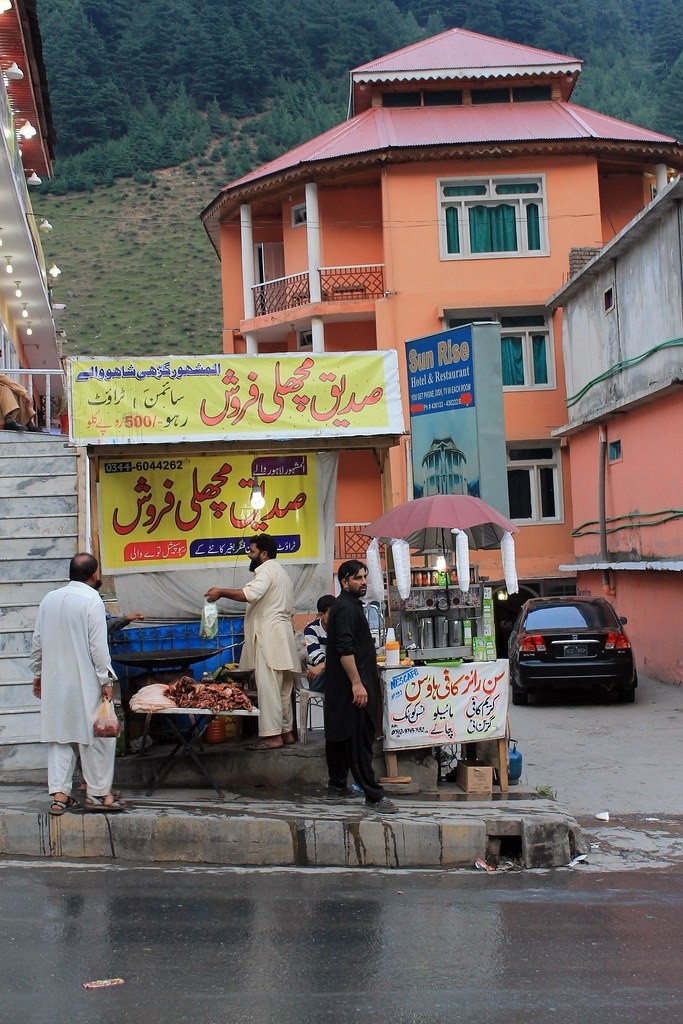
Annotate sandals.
[82,793,128,812]
[48,796,81,816]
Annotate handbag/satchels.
[198,597,220,640]
[92,698,122,738]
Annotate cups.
[385,641,400,665]
[455,535,469,592]
[386,627,395,641]
[502,540,516,592]
[367,550,384,600]
[391,545,411,599]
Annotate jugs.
[448,619,463,647]
[434,615,448,647]
[419,617,435,649]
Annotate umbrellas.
[354,495,520,551]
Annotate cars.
[507,596,638,705]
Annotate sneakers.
[364,796,400,814]
[326,784,359,800]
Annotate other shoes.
[77,780,120,800]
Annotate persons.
[202,533,303,750]
[30,553,150,816]
[301,559,401,814]
[0,374,50,433]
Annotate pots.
[112,646,222,668]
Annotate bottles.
[399,646,406,661]
[205,715,226,744]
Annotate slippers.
[248,738,284,750]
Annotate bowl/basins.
[224,668,255,679]
